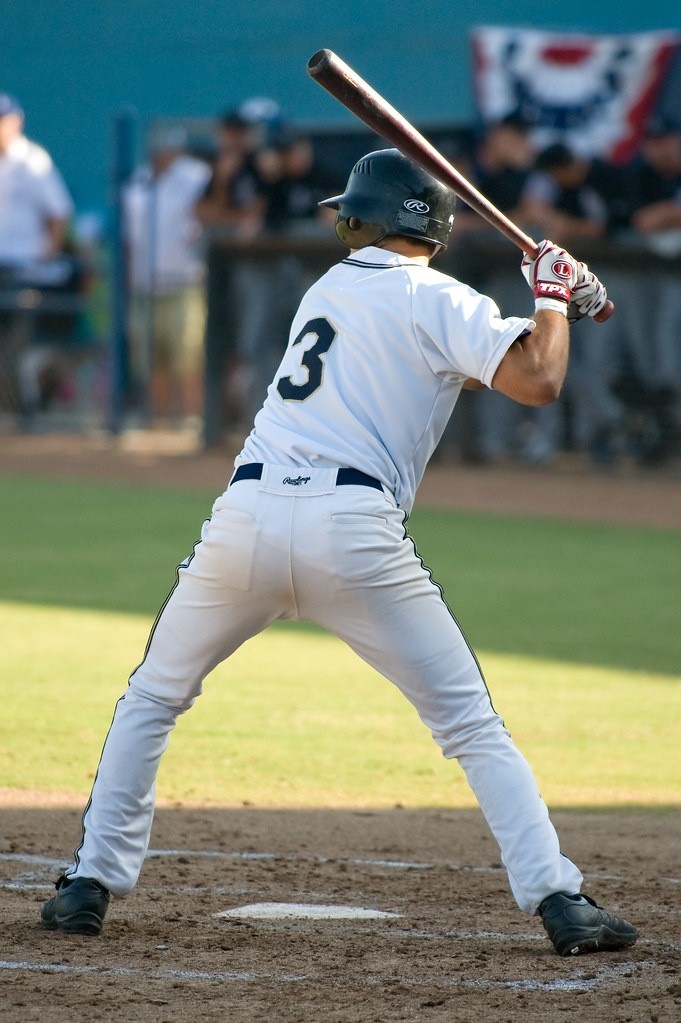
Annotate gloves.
[522,239,607,325]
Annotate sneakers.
[538,893,638,956]
[40,875,110,936]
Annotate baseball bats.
[310,50,618,327]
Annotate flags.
[470,23,681,168]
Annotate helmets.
[318,147,456,258]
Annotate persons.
[198,97,336,448]
[32,149,641,954]
[0,92,96,431]
[121,133,213,455]
[451,116,681,474]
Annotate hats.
[0,95,25,121]
